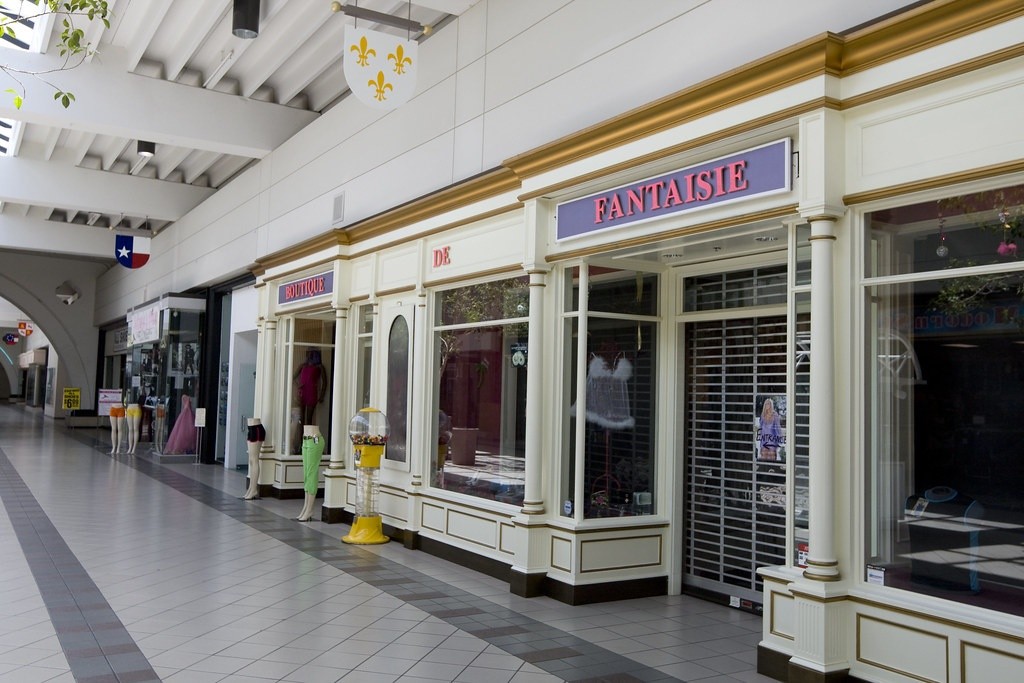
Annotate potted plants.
[447,359,491,465]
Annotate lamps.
[232,0,261,39]
[137,140,156,157]
[1004,223,1012,245]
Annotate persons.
[293,346,327,454]
[144,355,149,372]
[137,381,155,405]
[162,395,197,455]
[125,361,131,389]
[241,418,266,500]
[295,425,325,521]
[126,403,142,455]
[110,403,125,454]
[183,344,195,375]
[155,404,165,454]
[758,399,783,460]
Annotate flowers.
[591,495,608,518]
